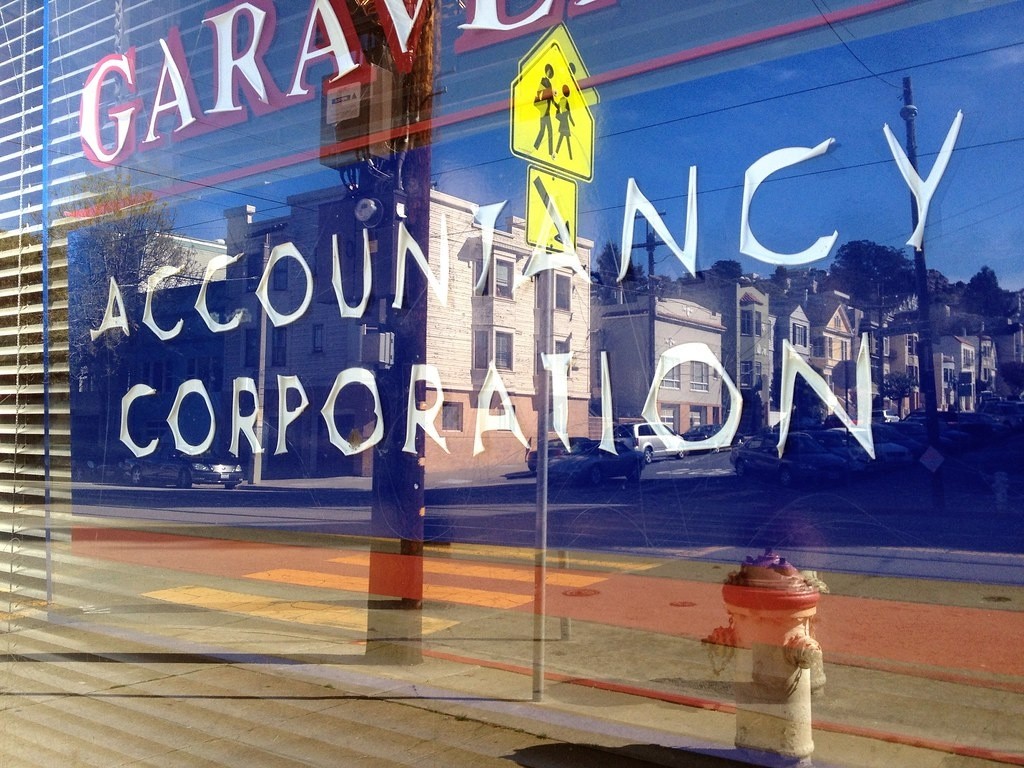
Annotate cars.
[524,389,1024,498]
[117,438,246,490]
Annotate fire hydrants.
[701,542,834,768]
